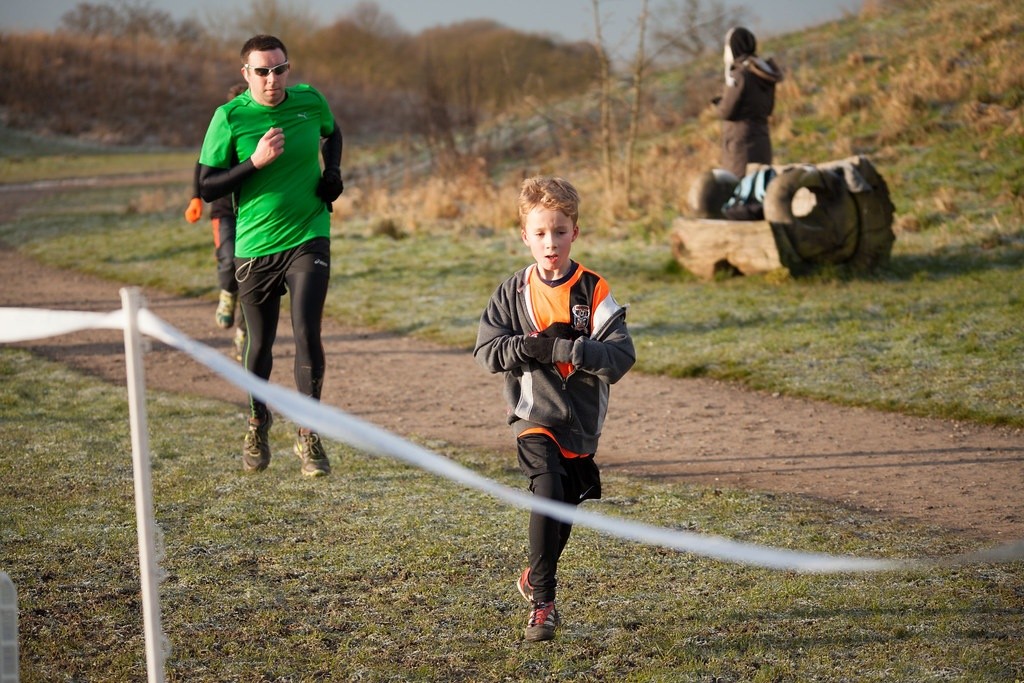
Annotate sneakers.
[293,427,336,478]
[216,289,237,329]
[524,598,559,642]
[232,326,246,360]
[243,409,272,473]
[516,568,560,626]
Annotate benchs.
[670,167,859,280]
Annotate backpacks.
[720,163,778,221]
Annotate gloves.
[537,322,578,341]
[315,174,344,213]
[524,333,555,364]
[186,198,205,223]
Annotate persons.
[707,27,783,177]
[186,34,345,478]
[473,178,637,643]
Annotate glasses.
[245,59,289,77]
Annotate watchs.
[529,330,539,338]
